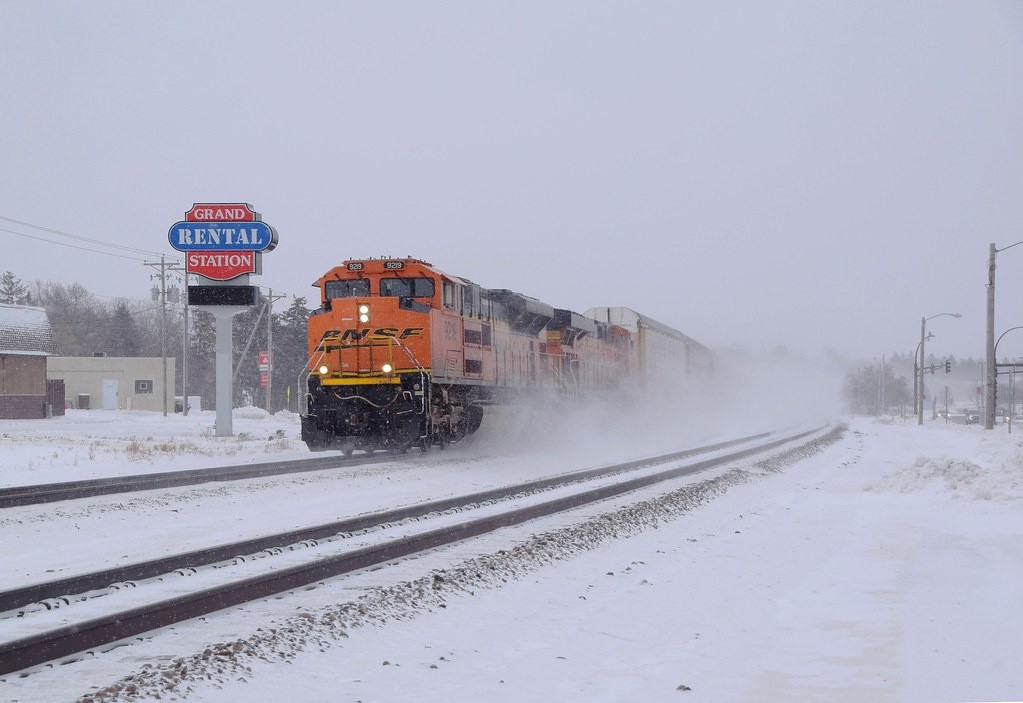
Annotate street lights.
[913,335,937,416]
[918,313,961,424]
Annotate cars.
[937,410,950,420]
[995,407,1007,423]
[175,398,191,413]
[1013,415,1023,424]
[966,410,979,423]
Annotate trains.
[299,255,715,456]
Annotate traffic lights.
[946,360,951,373]
